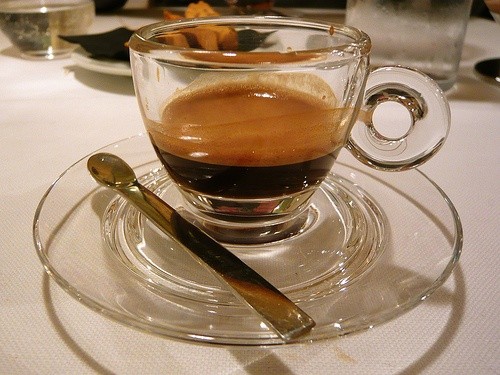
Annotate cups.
[346,0,473,92]
[126,15,451,227]
[0,0,96,60]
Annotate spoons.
[86,152,316,340]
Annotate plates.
[33,130,464,347]
[474,56,500,90]
[74,44,130,77]
